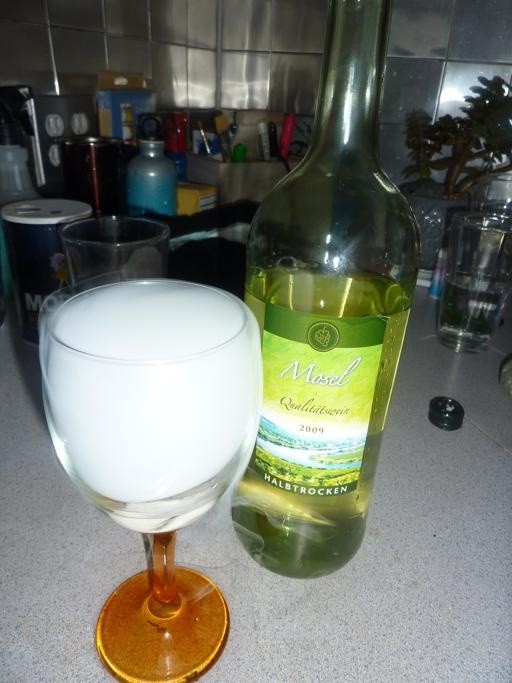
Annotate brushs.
[214,115,231,162]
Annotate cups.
[0,197,272,346]
[434,210,512,354]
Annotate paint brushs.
[197,121,211,154]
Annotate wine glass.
[34,273,271,681]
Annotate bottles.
[124,113,178,223]
[60,137,128,217]
[0,121,41,296]
[235,1,425,584]
